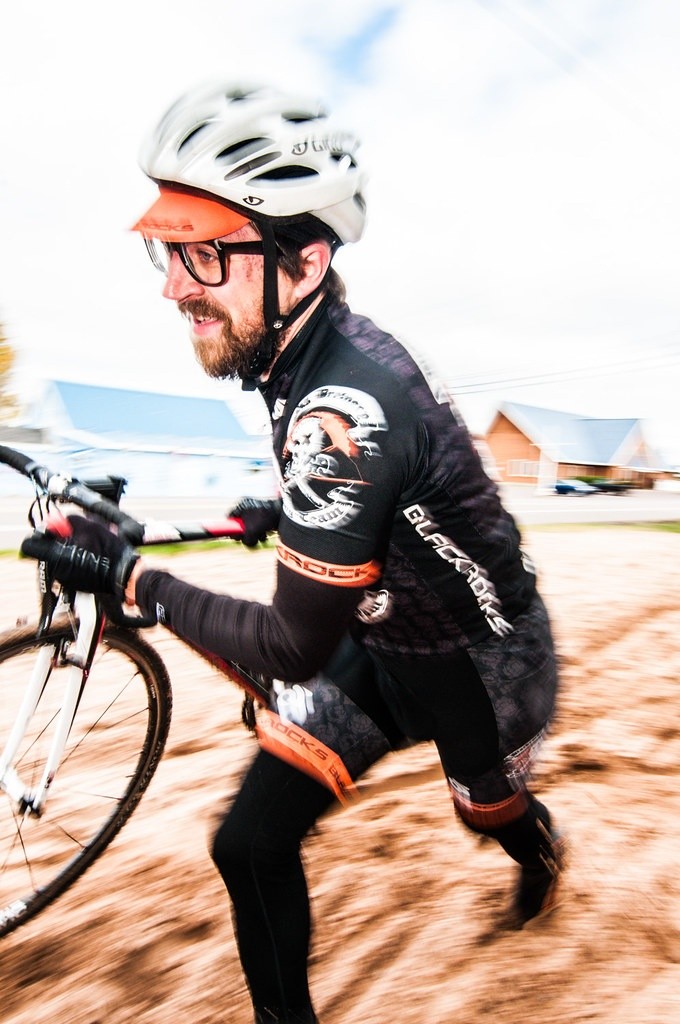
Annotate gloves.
[227,495,284,550]
[16,514,136,619]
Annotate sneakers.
[507,833,566,931]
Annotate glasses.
[141,235,285,288]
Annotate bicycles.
[1,435,287,941]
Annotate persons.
[21,76,565,1021]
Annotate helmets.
[136,72,368,246]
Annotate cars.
[555,478,640,497]
